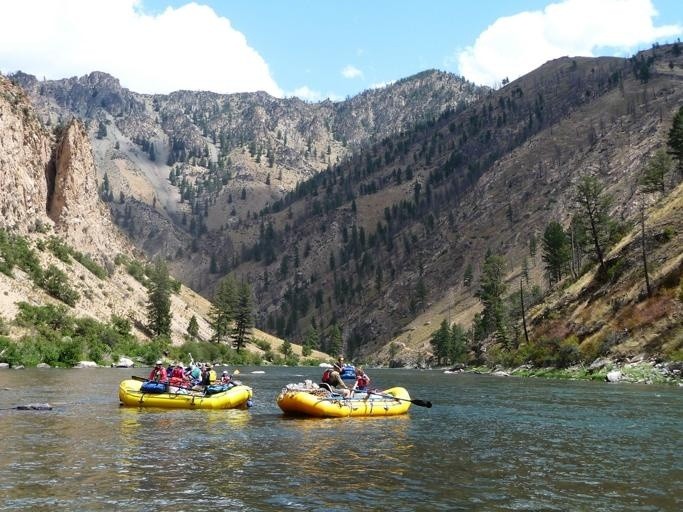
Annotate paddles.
[345,387,432,408]
[329,358,340,369]
[131,375,203,392]
[228,369,239,379]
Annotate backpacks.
[322,370,333,382]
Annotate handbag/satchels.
[141,381,165,393]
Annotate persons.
[322,361,348,391]
[147,359,231,393]
[348,368,372,393]
[333,354,346,375]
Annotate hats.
[156,361,162,365]
[204,363,213,368]
[334,362,342,370]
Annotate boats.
[338,364,356,379]
[119,378,253,409]
[278,385,412,417]
